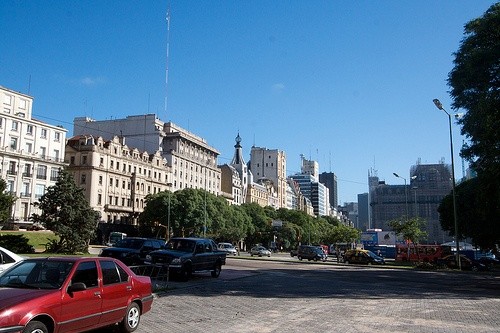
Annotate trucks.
[360,228,396,246]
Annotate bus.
[395,244,451,264]
[330,243,354,256]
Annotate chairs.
[46,268,60,284]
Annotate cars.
[437,254,500,273]
[290,246,300,257]
[0,257,155,333]
[342,248,386,266]
[0,247,37,288]
[97,235,168,276]
[217,243,237,256]
[246,246,271,258]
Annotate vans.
[297,245,327,262]
[108,232,127,246]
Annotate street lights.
[392,172,410,261]
[433,98,461,269]
[411,187,418,219]
[166,183,172,241]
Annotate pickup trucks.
[143,237,228,282]
